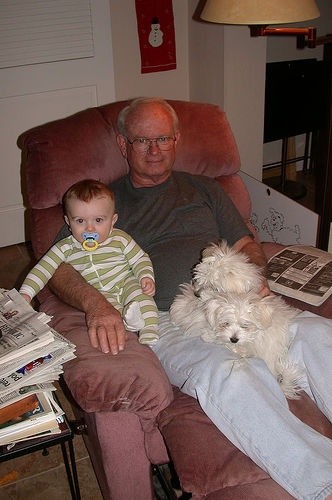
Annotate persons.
[48,99,332,500]
[19,180,159,344]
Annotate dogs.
[169,238,307,400]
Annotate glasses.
[127,136,177,152]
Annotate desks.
[0,391,88,500]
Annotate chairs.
[16,98,332,500]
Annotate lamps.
[200,0,332,49]
